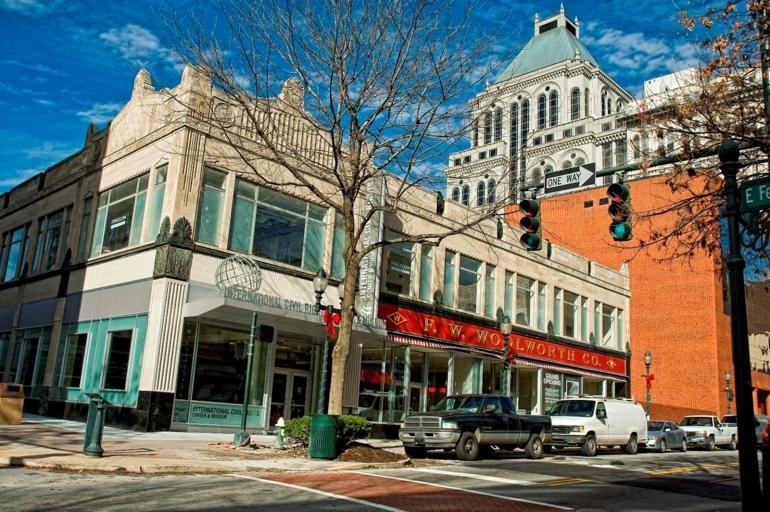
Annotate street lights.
[721,370,731,416]
[498,316,513,397]
[644,351,652,424]
[311,267,346,413]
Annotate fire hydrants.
[272,418,289,450]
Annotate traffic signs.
[740,176,770,207]
[544,161,597,192]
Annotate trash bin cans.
[0,382,26,424]
[310,413,340,459]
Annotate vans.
[545,398,649,456]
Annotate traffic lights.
[518,198,542,250]
[606,184,634,241]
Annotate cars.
[638,420,688,452]
[722,409,770,443]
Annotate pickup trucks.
[400,393,553,459]
[676,416,737,451]
